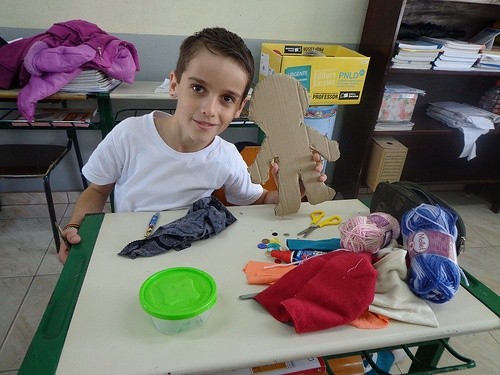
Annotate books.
[0,108,98,123]
[60,69,121,93]
[218,357,326,375]
[391,29,500,72]
[374,121,415,131]
[480,89,500,115]
[155,78,171,94]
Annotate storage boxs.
[364,136,407,192]
[377,93,418,123]
[258,43,370,104]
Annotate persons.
[59,27,327,265]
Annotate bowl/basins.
[139,267,217,336]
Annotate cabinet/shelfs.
[331,0,500,213]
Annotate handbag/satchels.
[370,180,466,256]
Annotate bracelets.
[64,224,80,229]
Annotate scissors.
[296,210,342,238]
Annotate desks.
[0,81,265,213]
[18,199,500,375]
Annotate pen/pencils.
[56,222,70,252]
[144,211,160,240]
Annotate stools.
[0,129,88,253]
